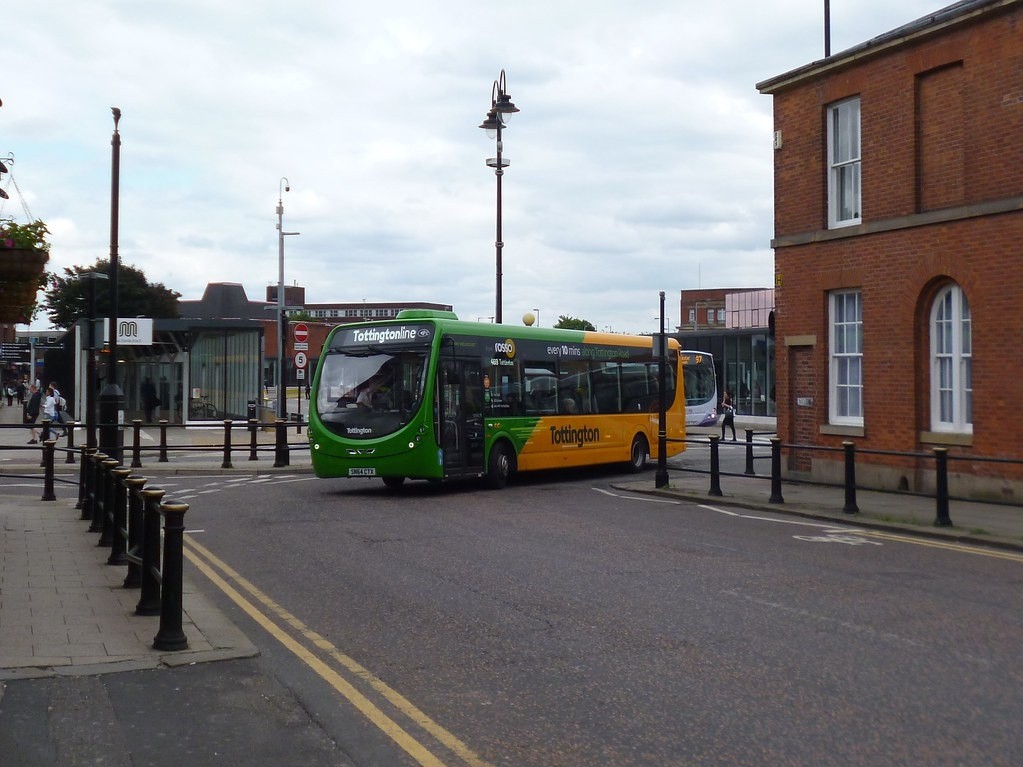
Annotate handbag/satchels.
[149,395,162,409]
[725,408,732,419]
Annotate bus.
[680,350,719,429]
[307,305,688,490]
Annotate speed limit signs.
[293,350,308,369]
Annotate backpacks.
[59,397,67,411]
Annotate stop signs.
[294,322,309,343]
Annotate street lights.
[477,69,521,323]
[274,176,301,419]
[98,106,125,460]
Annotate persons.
[305,383,310,400]
[140,378,156,423]
[7,385,14,406]
[35,375,40,386]
[741,378,748,404]
[754,380,761,398]
[23,374,29,384]
[41,388,60,440]
[357,380,393,409]
[27,384,43,444]
[14,380,25,405]
[48,382,68,437]
[719,392,737,441]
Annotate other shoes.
[720,438,725,441]
[39,432,43,442]
[731,439,737,441]
[27,439,37,444]
[55,432,59,440]
[59,430,68,437]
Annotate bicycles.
[177,394,218,421]
